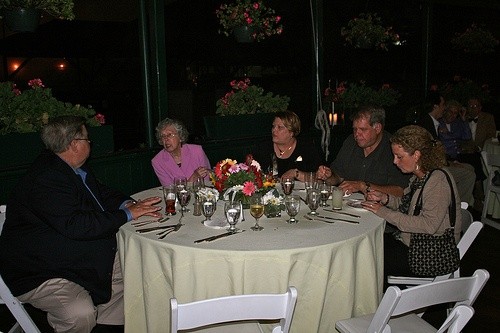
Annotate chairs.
[0,204,44,333]
[335,269,490,333]
[481,151,500,230]
[170,287,296,333]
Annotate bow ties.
[469,118,478,123]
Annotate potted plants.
[0,0,76,34]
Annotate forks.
[130,217,170,227]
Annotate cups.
[332,186,343,211]
[165,187,176,215]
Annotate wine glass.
[163,176,217,224]
[250,196,265,232]
[282,171,331,224]
[224,201,240,231]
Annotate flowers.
[216,73,291,116]
[216,0,283,43]
[327,81,401,108]
[207,156,277,195]
[0,76,105,135]
[340,12,408,49]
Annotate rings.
[370,205,372,206]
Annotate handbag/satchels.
[408,168,461,277]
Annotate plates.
[346,199,369,211]
[328,190,353,199]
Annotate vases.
[227,189,266,209]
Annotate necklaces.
[276,143,294,157]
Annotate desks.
[481,137,500,226]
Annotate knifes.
[323,209,361,218]
[207,229,245,241]
[136,224,178,233]
[318,216,361,224]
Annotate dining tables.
[116,178,386,333]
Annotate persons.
[361,125,463,292]
[315,105,410,242]
[255,110,327,192]
[417,90,497,213]
[151,118,212,186]
[1,114,162,333]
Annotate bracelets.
[124,201,137,207]
[365,180,371,193]
[295,168,299,179]
[381,194,389,206]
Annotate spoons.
[160,223,182,239]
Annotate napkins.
[203,215,236,231]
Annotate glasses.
[75,136,95,144]
[160,131,177,139]
[468,104,480,108]
